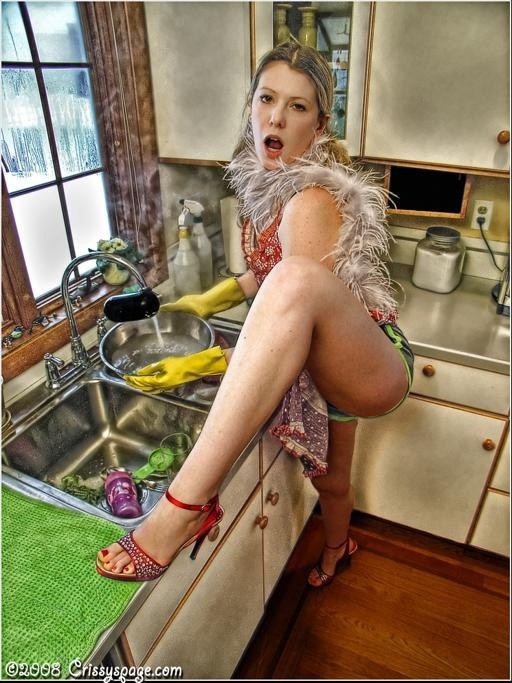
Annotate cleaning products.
[171,212,203,297]
[178,196,214,292]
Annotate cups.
[159,432,194,486]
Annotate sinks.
[2,368,216,524]
[103,315,240,412]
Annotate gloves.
[123,345,229,396]
[159,276,249,321]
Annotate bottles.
[273,3,317,48]
[174,226,200,297]
[104,468,143,520]
[411,226,466,293]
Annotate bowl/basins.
[100,310,215,380]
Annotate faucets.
[61,251,160,363]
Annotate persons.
[94,39,414,589]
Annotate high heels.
[94,490,224,581]
[306,537,358,587]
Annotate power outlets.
[470,200,494,230]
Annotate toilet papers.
[220,195,249,275]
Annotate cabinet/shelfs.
[110,411,321,679]
[469,427,511,558]
[143,1,510,173]
[349,355,510,545]
